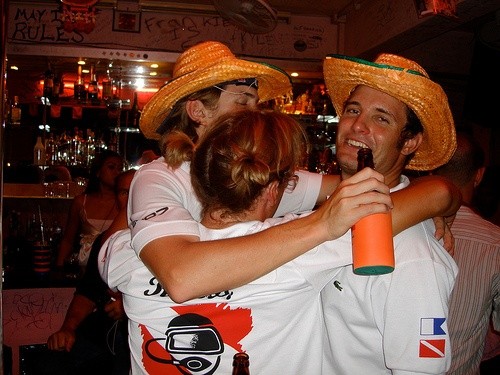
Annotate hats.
[324,53,457,170]
[139,41,292,140]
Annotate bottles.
[10,96,21,125]
[73,69,113,106]
[350,148,395,276]
[130,92,139,126]
[32,126,108,169]
[3,204,62,275]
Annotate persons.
[319,54,458,375]
[127,41,393,304]
[47,169,136,375]
[53,149,122,283]
[98,107,464,375]
[428,131,500,375]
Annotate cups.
[33,240,51,273]
[64,253,80,278]
[40,175,89,199]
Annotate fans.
[213,0,278,35]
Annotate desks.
[1,176,87,236]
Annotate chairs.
[9,164,44,250]
[44,166,71,247]
